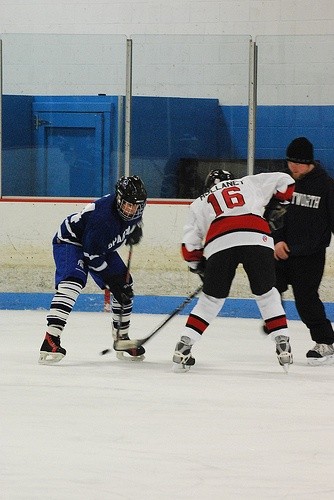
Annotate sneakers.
[275,334,293,375]
[113,337,145,361]
[39,331,66,366]
[171,336,196,373]
[305,343,334,366]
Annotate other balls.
[101,349,110,355]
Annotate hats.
[286,137,314,164]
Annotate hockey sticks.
[112,283,204,351]
[115,243,145,363]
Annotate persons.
[261,136,334,357]
[171,169,295,366]
[39,175,148,356]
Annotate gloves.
[107,275,131,305]
[188,255,206,274]
[125,223,143,246]
[265,200,290,232]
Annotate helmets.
[115,174,148,224]
[203,169,234,194]
